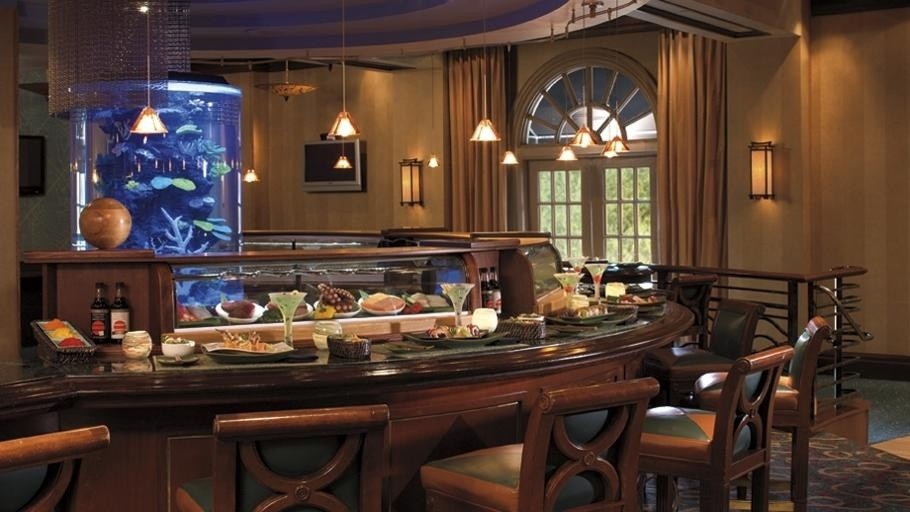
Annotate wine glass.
[552,254,610,315]
[268,291,307,353]
[440,281,474,338]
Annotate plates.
[156,356,201,367]
[215,291,408,325]
[602,295,668,307]
[203,341,320,365]
[400,325,511,350]
[547,311,636,327]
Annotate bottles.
[480,266,503,318]
[89,280,132,346]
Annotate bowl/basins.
[160,337,198,359]
[327,333,373,360]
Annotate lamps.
[551,1,630,162]
[129,2,169,133]
[468,1,502,142]
[400,157,424,206]
[325,1,362,138]
[748,139,775,200]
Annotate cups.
[469,308,499,335]
[604,282,626,301]
[121,329,154,362]
